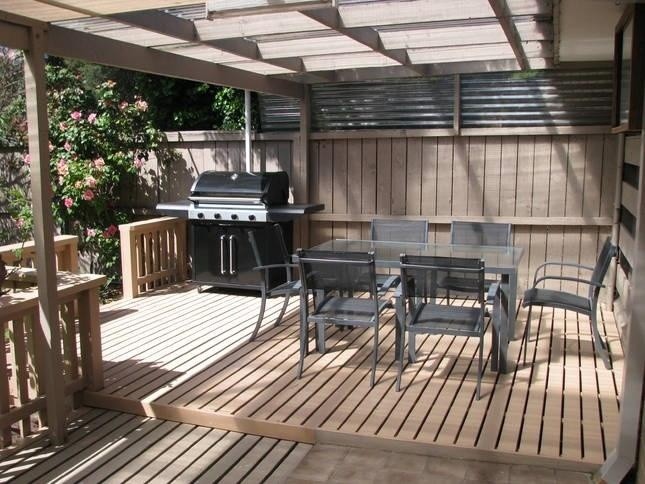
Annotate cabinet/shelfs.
[186,220,297,296]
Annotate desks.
[292,238,524,375]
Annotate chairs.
[350,218,429,304]
[391,253,500,401]
[436,220,512,299]
[247,223,330,344]
[296,247,392,387]
[522,236,617,370]
